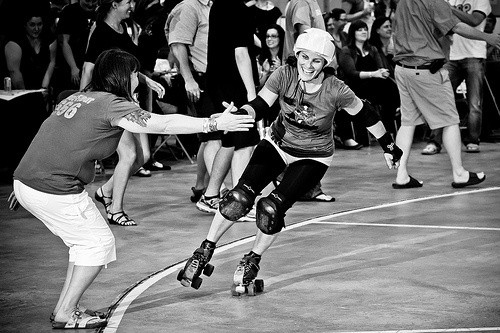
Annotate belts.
[395,59,430,70]
[192,70,206,77]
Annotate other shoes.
[421,143,440,155]
[313,193,336,202]
[133,169,152,177]
[464,143,480,153]
[342,138,364,150]
[104,169,114,177]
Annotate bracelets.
[368,71,372,79]
[203,117,208,133]
[209,116,218,131]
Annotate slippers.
[392,172,423,189]
[48,305,108,329]
[452,170,486,188]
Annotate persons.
[7,48,256,329]
[163,0,233,203]
[0,0,500,186]
[195,0,261,222]
[392,0,500,189]
[272,0,336,203]
[79,0,166,226]
[177,27,403,285]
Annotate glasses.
[266,34,279,39]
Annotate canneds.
[4,77,11,91]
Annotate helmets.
[293,28,336,69]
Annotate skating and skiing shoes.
[231,254,264,296]
[177,242,216,289]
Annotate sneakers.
[236,205,256,222]
[195,192,223,213]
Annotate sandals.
[107,210,137,226]
[190,187,206,202]
[95,186,113,214]
